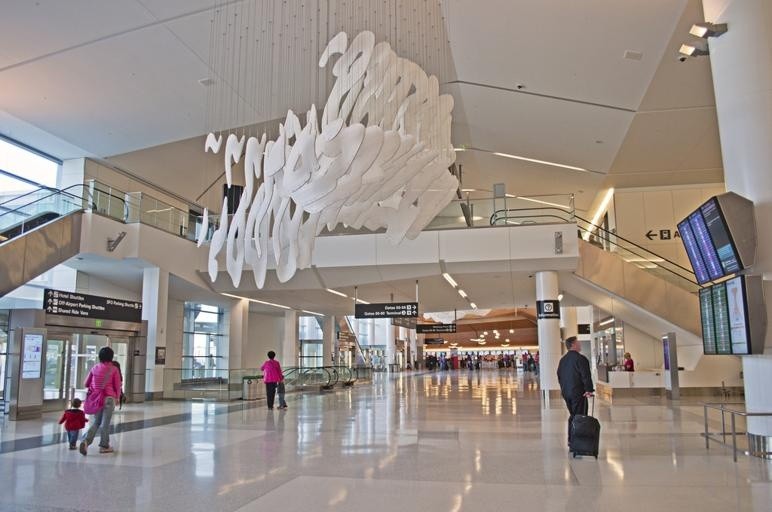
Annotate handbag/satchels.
[84,388,104,414]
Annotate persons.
[58,398,86,449]
[497,353,517,369]
[556,337,595,452]
[260,350,282,410]
[522,351,539,377]
[276,375,288,411]
[424,352,450,370]
[624,352,634,371]
[79,347,122,455]
[467,354,482,369]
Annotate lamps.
[680,22,727,56]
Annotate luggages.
[570,395,600,459]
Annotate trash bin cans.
[389,364,400,372]
[242,375,266,400]
[736,354,772,437]
[415,361,421,369]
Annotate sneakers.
[269,405,287,410]
[79,441,87,455]
[99,447,113,453]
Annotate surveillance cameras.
[679,56,688,63]
[517,85,522,89]
